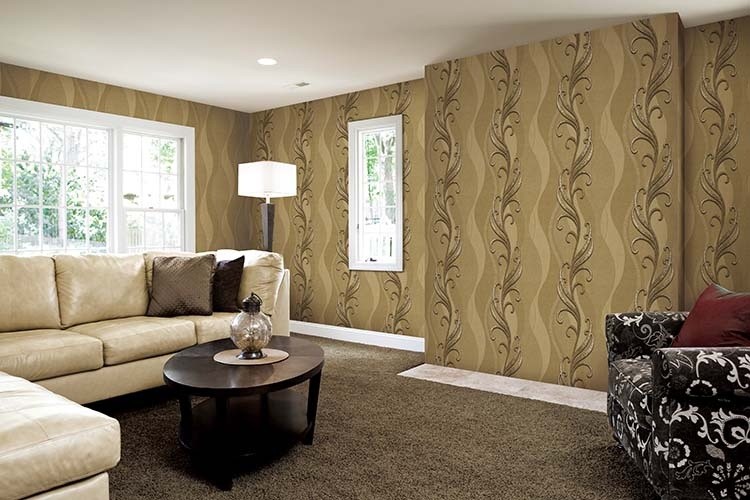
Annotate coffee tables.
[162,336,324,489]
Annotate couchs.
[605,311,750,500]
[0,248,290,500]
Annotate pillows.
[675,281,750,347]
[148,251,215,317]
[217,255,246,315]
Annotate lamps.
[237,160,296,253]
